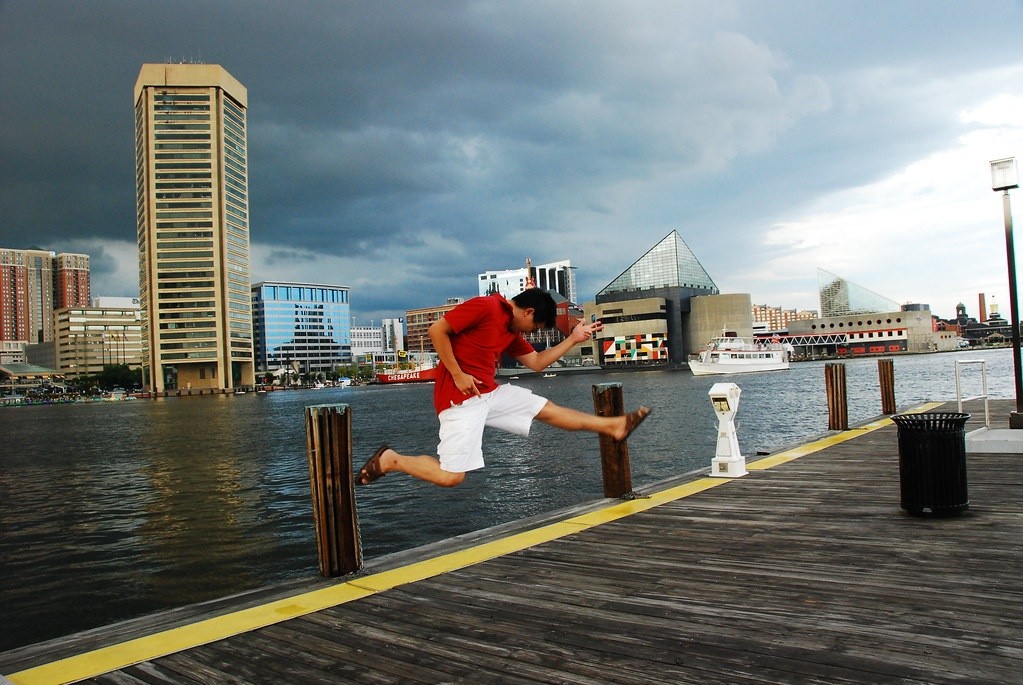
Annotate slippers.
[615,408,652,446]
[354,445,390,487]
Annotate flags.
[102,331,128,342]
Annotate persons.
[354,288,653,488]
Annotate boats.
[687,323,791,377]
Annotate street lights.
[989,156,1023,430]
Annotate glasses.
[531,321,540,334]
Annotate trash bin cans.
[889,412,973,515]
